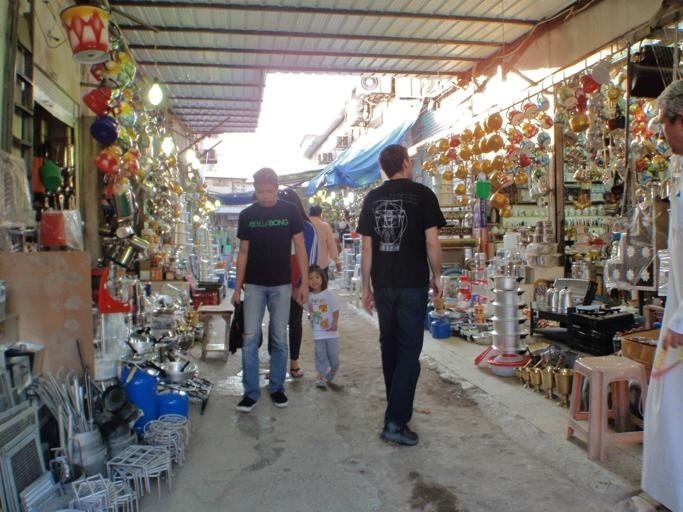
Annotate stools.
[567,356,648,462]
[69,413,191,511]
[197,306,233,360]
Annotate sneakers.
[271,391,289,407]
[237,396,256,412]
[316,378,327,389]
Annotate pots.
[489,276,529,355]
[87,367,137,437]
[125,327,198,383]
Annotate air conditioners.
[316,74,395,166]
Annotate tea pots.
[84,30,184,244]
[502,90,554,199]
[554,60,629,184]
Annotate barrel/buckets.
[215,269,225,283]
[72,425,103,451]
[227,267,236,288]
[78,446,109,478]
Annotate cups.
[97,224,150,268]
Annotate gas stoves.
[572,304,635,333]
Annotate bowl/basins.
[486,358,518,378]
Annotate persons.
[265,188,318,381]
[251,192,258,204]
[355,142,447,447]
[230,166,311,413]
[307,204,343,286]
[656,76,683,351]
[293,263,345,390]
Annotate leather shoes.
[381,420,417,445]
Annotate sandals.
[290,367,303,378]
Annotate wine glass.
[514,366,573,408]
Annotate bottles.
[546,286,573,314]
[204,283,224,305]
[571,249,600,280]
[25,226,39,253]
